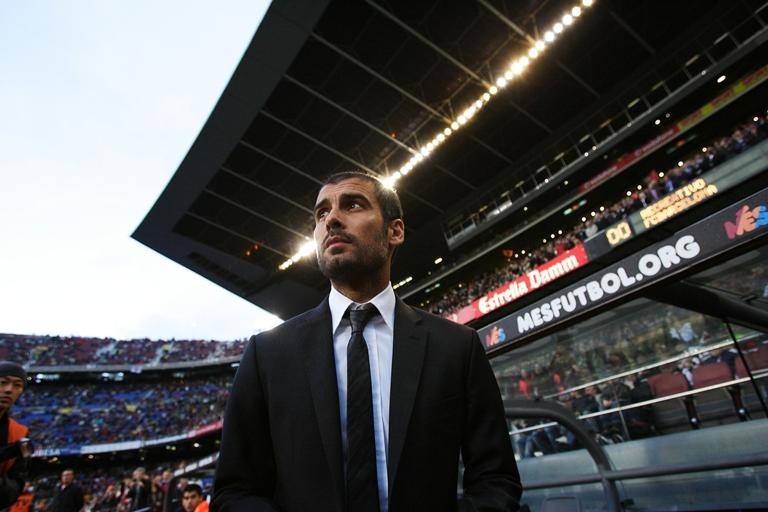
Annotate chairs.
[647,348,768,434]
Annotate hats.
[0,361,26,391]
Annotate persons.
[493,305,768,458]
[426,206,617,318]
[208,171,528,511]
[1,327,259,512]
[603,115,767,232]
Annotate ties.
[345,307,380,511]
